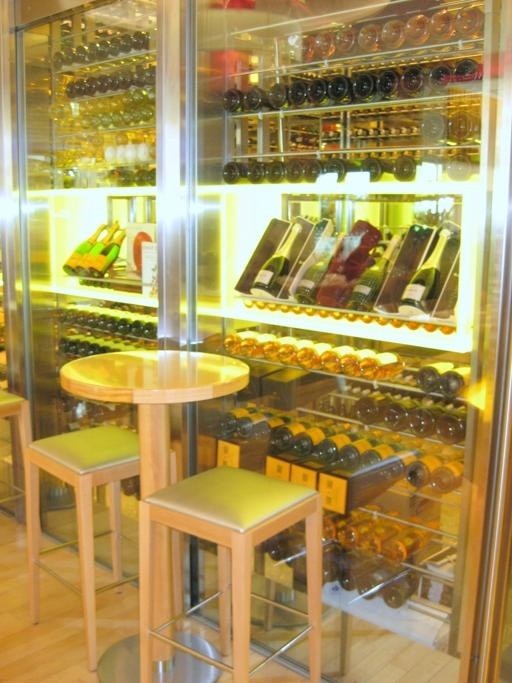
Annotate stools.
[25,425,181,673]
[137,466,324,682]
[1,391,44,551]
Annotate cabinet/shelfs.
[2,0,512,683]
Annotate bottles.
[248,222,455,312]
[58,305,467,610]
[51,5,485,188]
[64,223,126,278]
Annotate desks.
[59,350,252,683]
